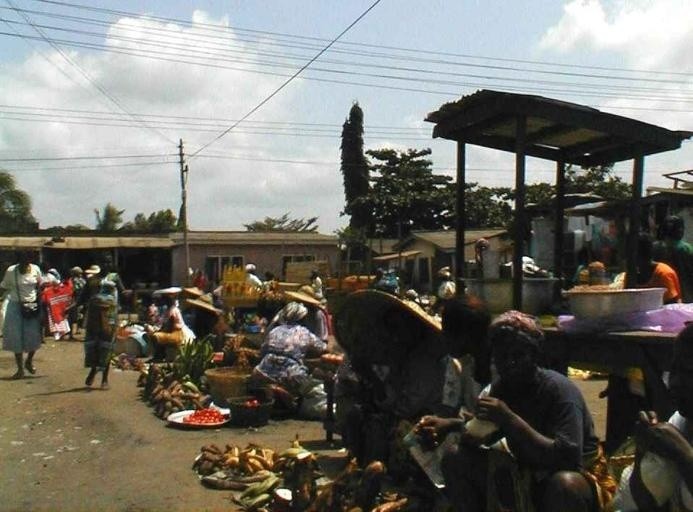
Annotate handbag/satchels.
[20,300,40,319]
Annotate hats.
[84,264,100,274]
[331,288,445,380]
[185,294,215,313]
[184,287,201,295]
[284,284,321,306]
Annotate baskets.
[203,364,252,407]
[226,394,276,428]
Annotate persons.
[0,217,692,512]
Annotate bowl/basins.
[458,275,562,317]
[558,286,670,322]
